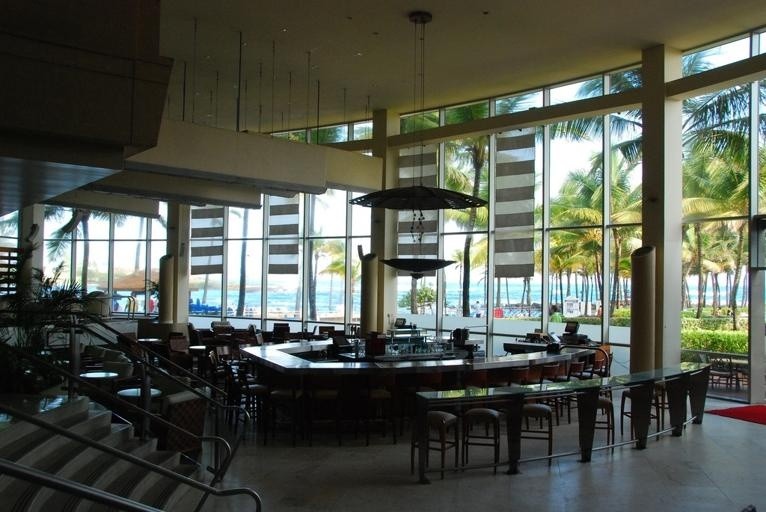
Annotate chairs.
[79,322,613,452]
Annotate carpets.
[704,404,766,425]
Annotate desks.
[416,361,712,485]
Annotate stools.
[411,384,686,479]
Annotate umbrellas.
[183,276,261,316]
[94,269,199,315]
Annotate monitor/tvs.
[565,322,579,333]
[394,318,406,327]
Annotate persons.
[143,291,155,314]
[549,305,564,322]
[472,301,482,317]
[597,305,602,317]
[124,292,139,313]
